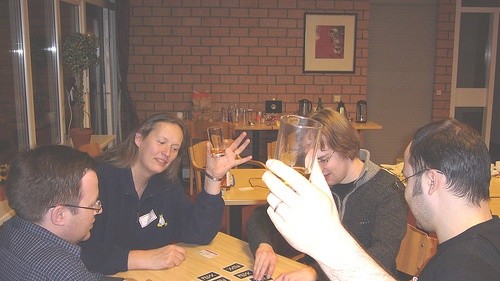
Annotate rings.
[274,201,283,212]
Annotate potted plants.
[63,34,100,148]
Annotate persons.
[0,145,105,281]
[78,113,252,275]
[247,109,409,281]
[262,118,500,281]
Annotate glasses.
[395,169,443,192]
[317,150,337,166]
[52,200,103,214]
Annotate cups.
[220,104,282,125]
[271,115,322,193]
[207,126,225,158]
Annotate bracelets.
[205,171,222,181]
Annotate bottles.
[222,170,230,191]
[356,100,367,122]
[317,98,323,110]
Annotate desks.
[105,231,309,281]
[216,118,383,169]
[217,168,500,241]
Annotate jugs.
[335,101,347,119]
[299,99,313,116]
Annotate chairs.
[188,120,277,196]
[393,223,438,276]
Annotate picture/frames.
[303,12,357,73]
